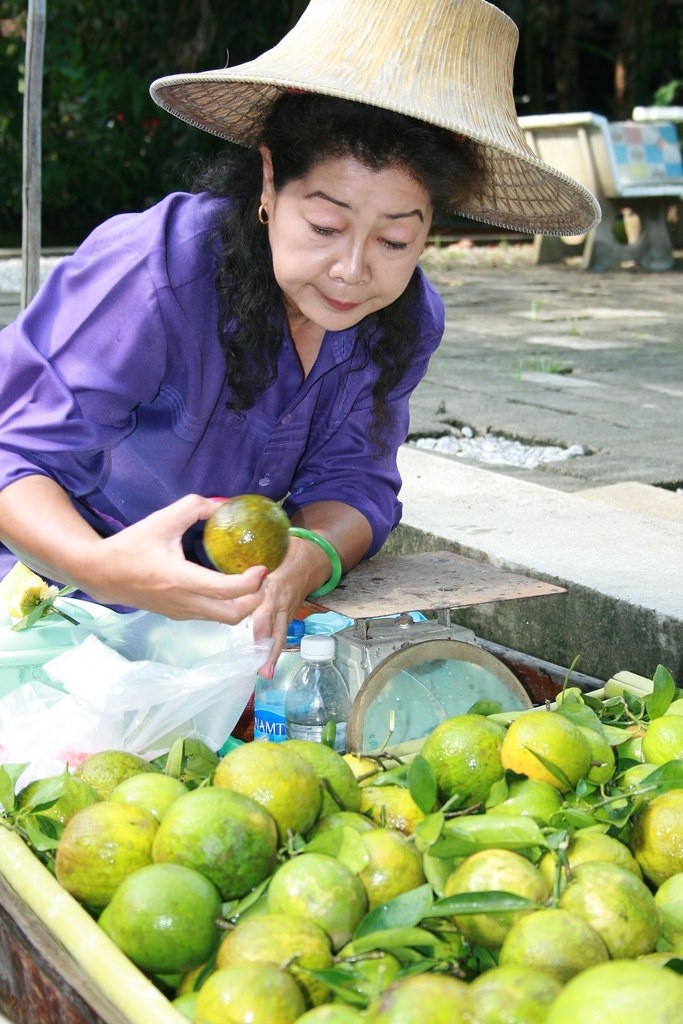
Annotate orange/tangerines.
[202,494,289,576]
[13,697,683,1024]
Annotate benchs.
[518,112,683,272]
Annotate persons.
[0,82,489,678]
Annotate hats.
[149,0,602,238]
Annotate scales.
[304,550,568,755]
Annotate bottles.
[285,634,350,756]
[253,619,304,745]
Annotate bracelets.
[288,526,342,598]
